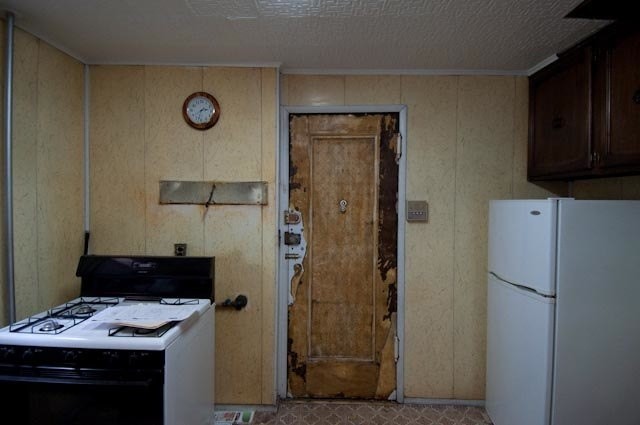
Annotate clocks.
[182,91,220,130]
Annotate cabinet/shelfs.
[528,45,638,184]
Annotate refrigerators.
[485,195,639,424]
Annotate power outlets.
[175,244,185,256]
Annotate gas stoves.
[0,295,216,351]
